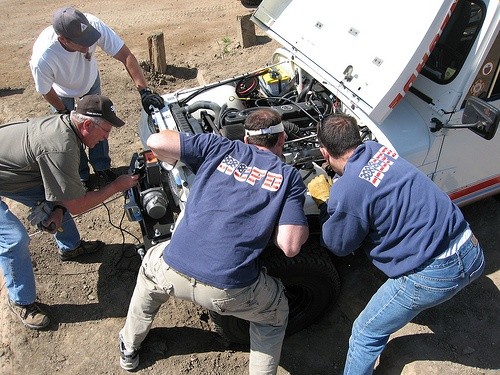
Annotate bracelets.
[52,205,67,216]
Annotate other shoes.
[94,172,105,191]
[80,178,90,191]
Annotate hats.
[52,7,101,46]
[74,95,125,127]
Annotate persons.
[0,93,139,328]
[29,7,165,193]
[119,107,309,375]
[307,112,485,375]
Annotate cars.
[124,0,500,345]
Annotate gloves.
[139,87,164,116]
[307,174,333,207]
[28,201,50,225]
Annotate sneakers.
[8,299,50,329]
[61,241,102,260]
[120,341,139,370]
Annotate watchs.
[139,87,153,96]
